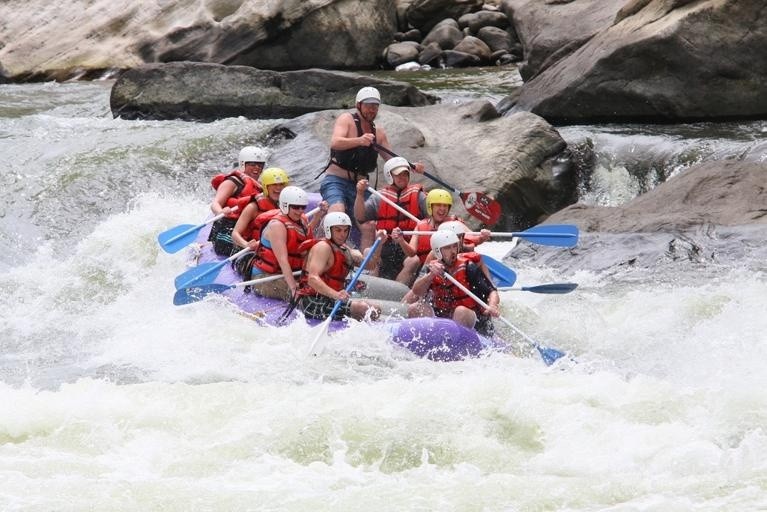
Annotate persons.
[354,158,498,338]
[313,86,425,260]
[209,146,387,322]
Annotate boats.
[188,214,510,364]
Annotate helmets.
[323,212,352,243]
[238,146,266,173]
[279,186,308,215]
[355,87,381,110]
[384,157,411,186]
[430,230,460,260]
[261,167,287,197]
[426,189,452,217]
[438,221,465,246]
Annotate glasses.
[246,162,265,166]
[289,205,307,209]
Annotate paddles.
[159,192,263,254]
[174,240,260,290]
[302,229,387,356]
[367,186,516,287]
[442,270,579,371]
[497,282,578,294]
[372,141,501,224]
[400,224,578,247]
[173,269,302,305]
